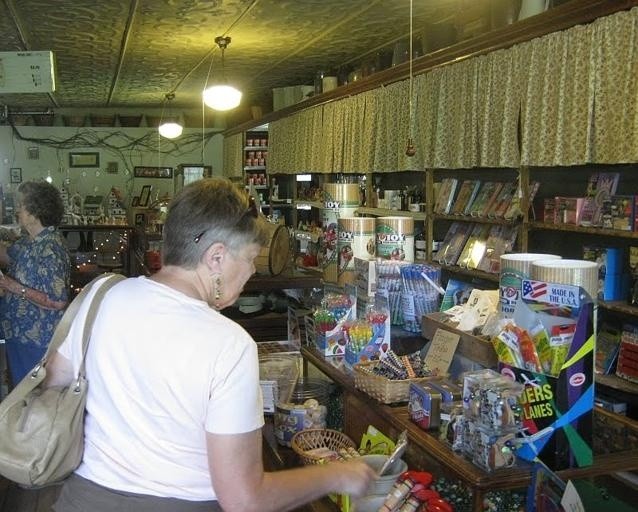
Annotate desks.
[57,224,134,278]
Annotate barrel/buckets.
[252,222,291,275]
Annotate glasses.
[195,195,259,243]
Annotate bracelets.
[18,286,26,301]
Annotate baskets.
[354,359,444,404]
[292,427,356,466]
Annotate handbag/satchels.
[1,364,87,489]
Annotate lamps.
[158,93,184,139]
[202,35,243,111]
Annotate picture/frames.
[10,151,173,207]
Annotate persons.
[43,174,381,512]
[0,181,72,388]
